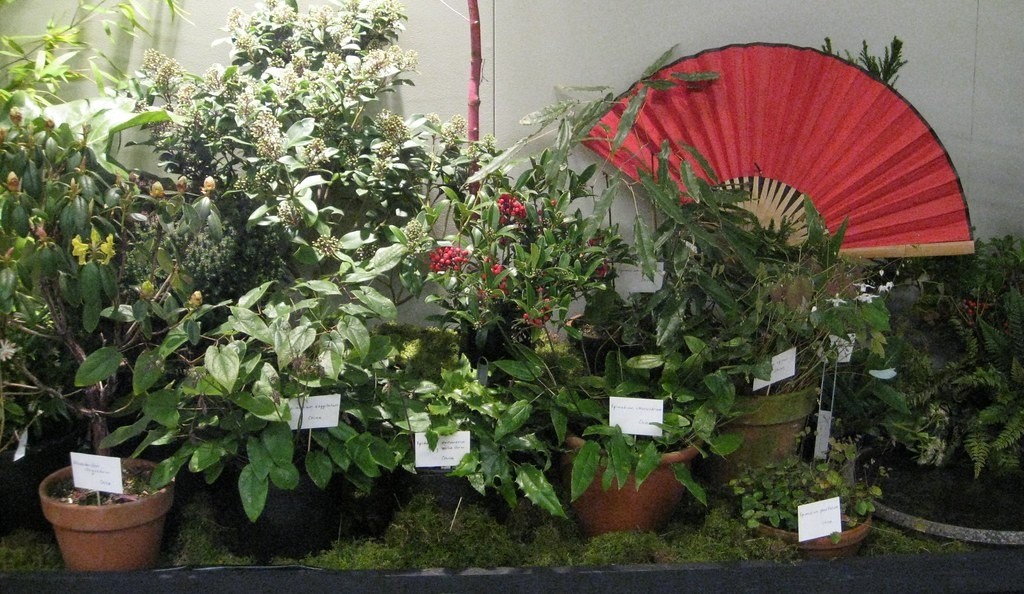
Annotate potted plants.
[0,1,1024,576]
